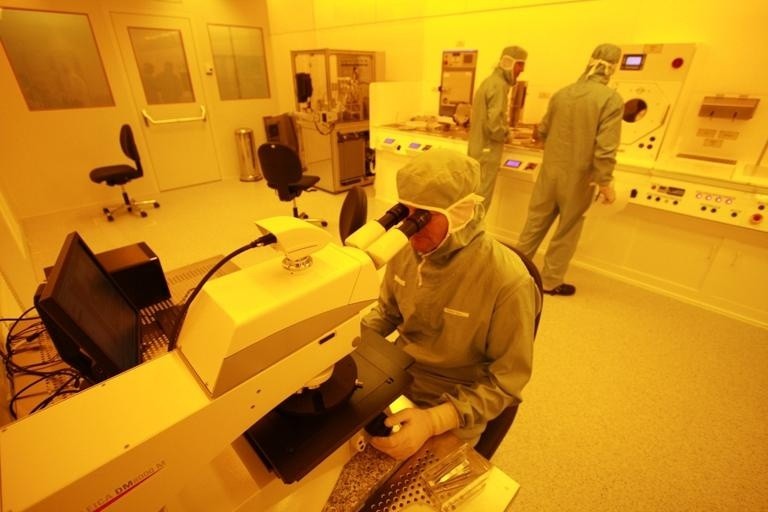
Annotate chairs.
[257,143,330,229]
[89,121,161,222]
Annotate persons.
[362,147,536,461]
[467,44,529,209]
[519,44,626,296]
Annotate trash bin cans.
[235,128,263,182]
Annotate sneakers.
[543,283,576,297]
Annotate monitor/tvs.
[34,230,142,385]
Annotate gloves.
[368,401,461,463]
